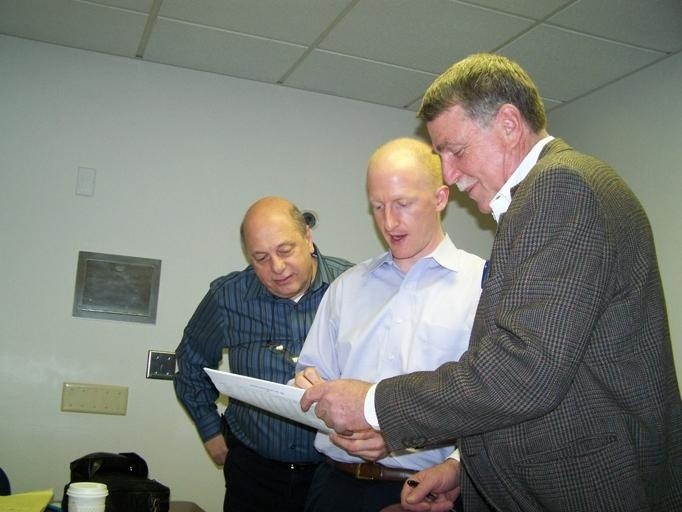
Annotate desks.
[47,497,210,511]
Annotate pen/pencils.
[407,480,457,512]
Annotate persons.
[282,136,495,511]
[296,46,681,509]
[170,195,358,512]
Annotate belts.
[274,459,302,472]
[324,460,417,482]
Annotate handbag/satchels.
[62,452,170,512]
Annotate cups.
[65,482,110,512]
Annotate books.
[200,365,338,436]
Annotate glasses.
[268,342,301,367]
